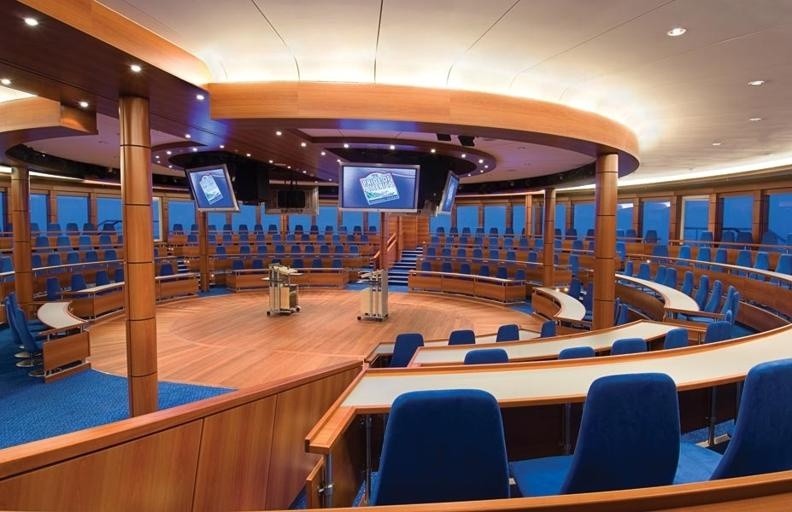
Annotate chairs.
[170,223,380,293]
[0,221,199,384]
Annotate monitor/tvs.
[435,169,460,216]
[185,164,239,212]
[265,187,319,215]
[337,162,421,213]
